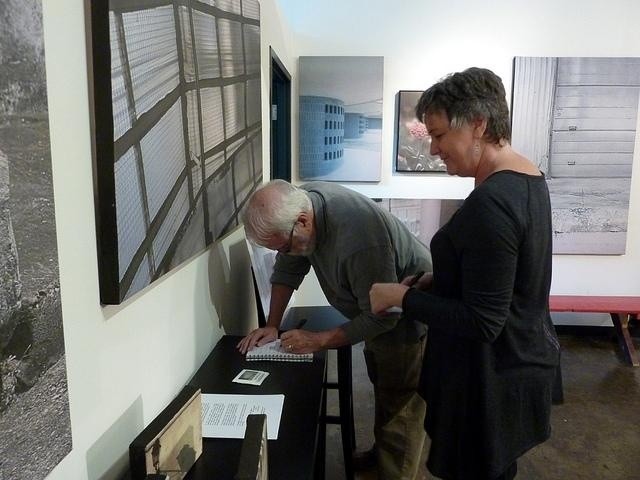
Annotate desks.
[125,334,328,480]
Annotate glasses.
[276,219,299,253]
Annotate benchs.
[549,296,640,366]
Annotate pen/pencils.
[279,318,307,350]
[409,272,425,287]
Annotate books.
[246,338,314,363]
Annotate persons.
[235,178,434,479]
[369,66,566,480]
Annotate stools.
[280,305,357,458]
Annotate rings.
[288,345,292,348]
